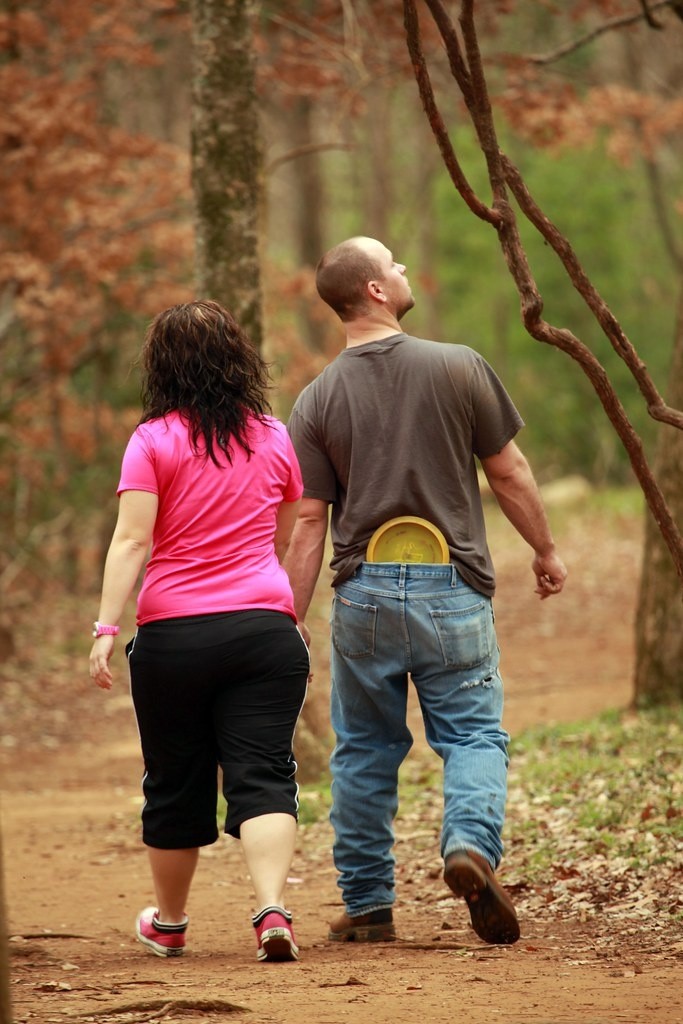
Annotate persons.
[282,235,568,944]
[89,300,311,958]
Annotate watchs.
[93,621,119,638]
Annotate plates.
[367,513,449,566]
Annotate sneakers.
[250,908,299,962]
[137,904,189,960]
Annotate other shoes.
[443,848,521,946]
[328,906,397,942]
